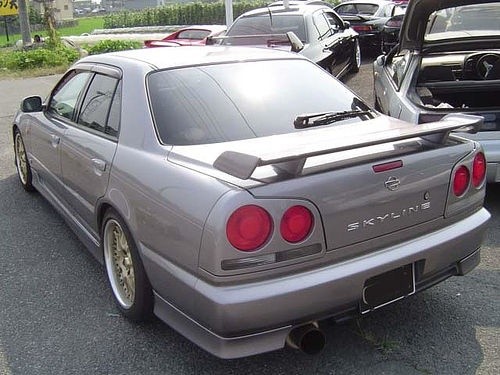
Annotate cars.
[12,45,492,358]
[373,1,500,184]
[145,25,231,48]
[332,1,406,56]
[218,5,361,81]
[268,0,333,9]
